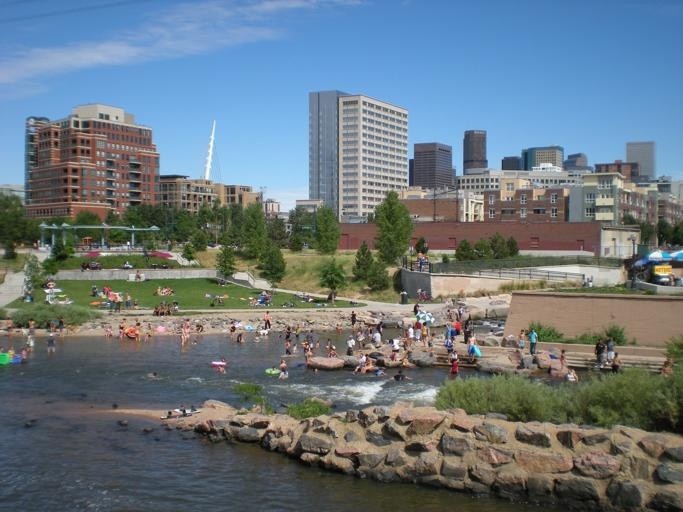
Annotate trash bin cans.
[400,292,408,304]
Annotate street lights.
[631,233,637,289]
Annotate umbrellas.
[643,248,672,264]
[670,251,683,262]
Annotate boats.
[175,409,200,413]
[211,361,225,367]
[265,368,280,375]
[126,327,137,338]
[0,353,22,366]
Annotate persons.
[417,250,423,262]
[660,357,673,376]
